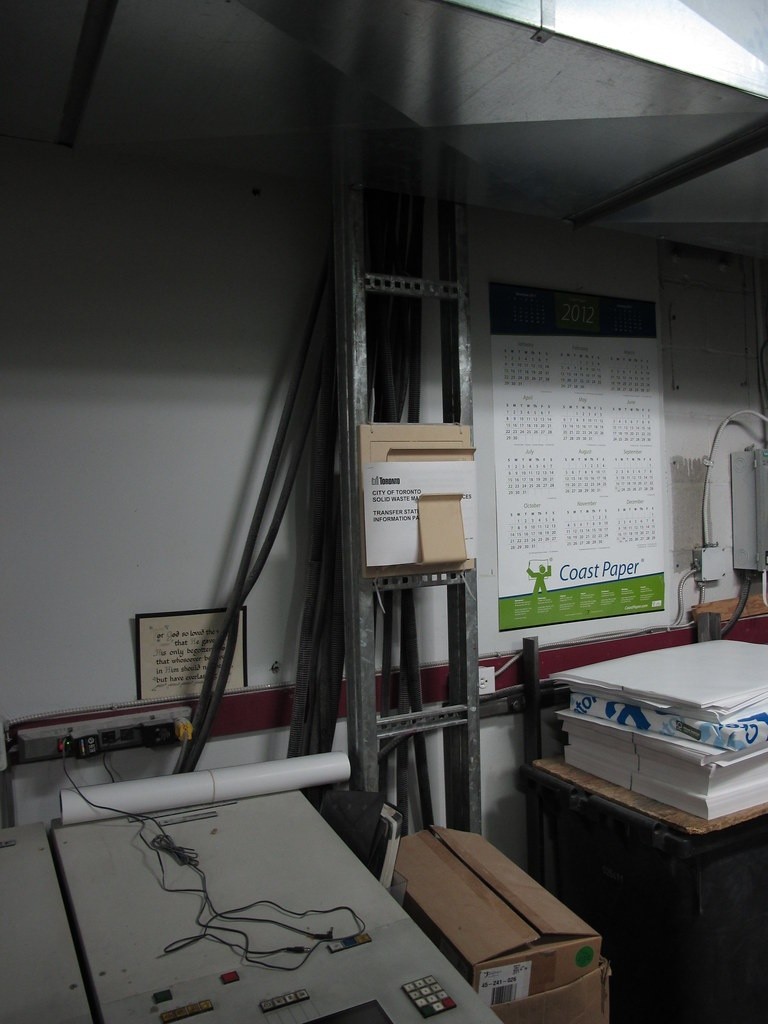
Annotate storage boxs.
[393,826,603,1005]
[490,955,612,1024]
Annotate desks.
[515,755,768,1024]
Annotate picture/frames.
[135,606,248,700]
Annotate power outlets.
[479,666,495,695]
[16,706,192,764]
